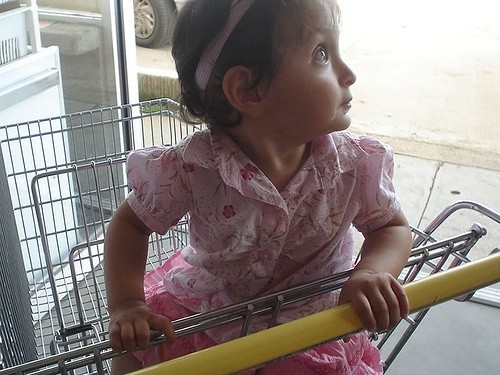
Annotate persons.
[103,1,411,375]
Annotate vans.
[17,1,189,49]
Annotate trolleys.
[0,98,500,375]
